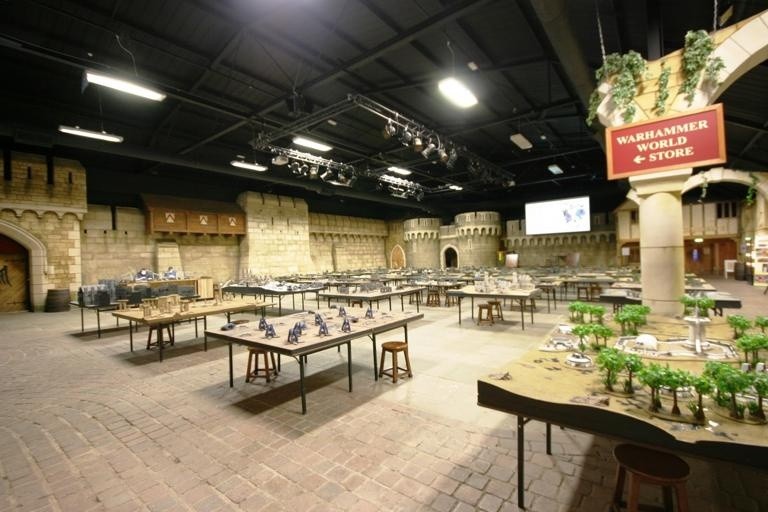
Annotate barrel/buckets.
[567,252,579,266]
[496,250,506,267]
[622,247,630,257]
[505,254,518,268]
[724,259,737,273]
[734,263,746,280]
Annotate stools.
[605,442,693,512]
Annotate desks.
[475,310,767,510]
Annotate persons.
[134,267,159,281]
[165,267,178,278]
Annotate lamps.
[256,93,520,200]
[81,31,168,103]
[56,85,128,146]
[438,38,483,112]
